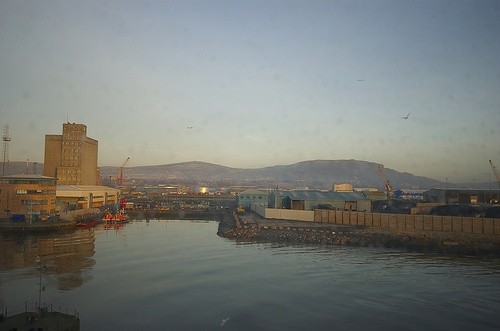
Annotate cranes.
[117,157,130,192]
[379,167,393,201]
[489,159,500,187]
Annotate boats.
[75,207,131,230]
